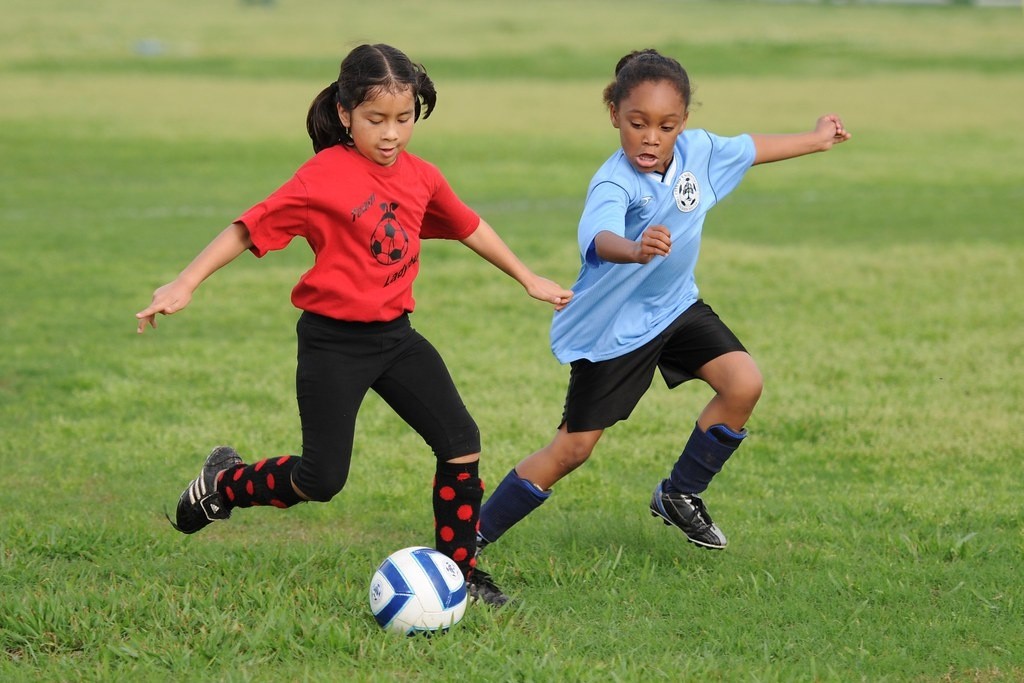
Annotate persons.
[136,43,573,606]
[477,49,851,554]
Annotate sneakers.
[175,446,242,535]
[464,564,508,605]
[648,478,727,553]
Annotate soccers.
[369,545,469,644]
